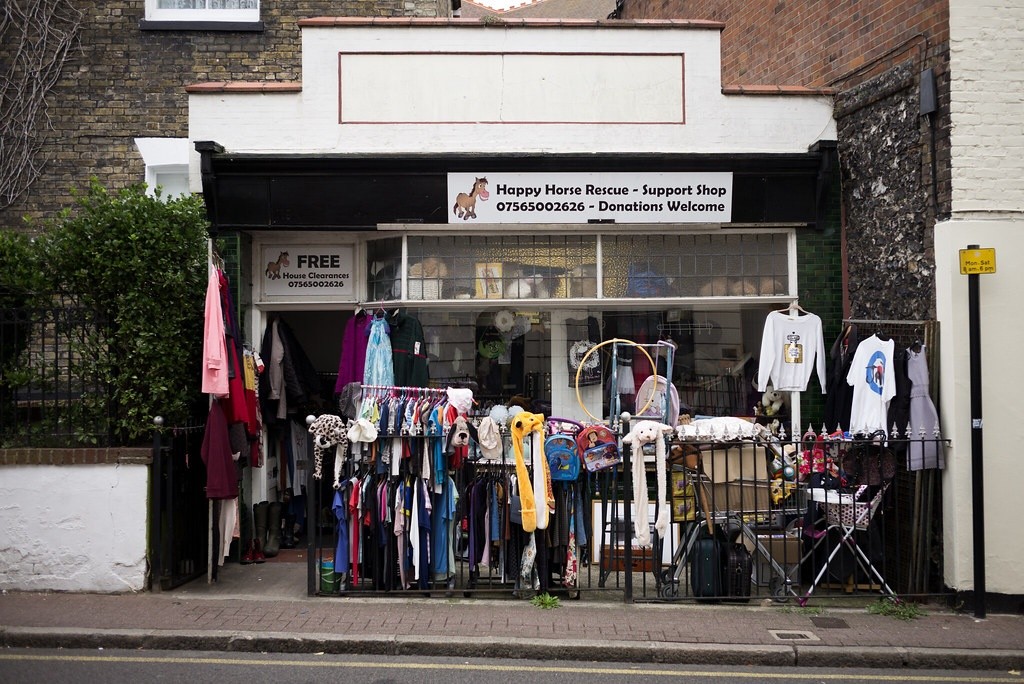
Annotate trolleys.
[651,436,793,604]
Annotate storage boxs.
[701,447,769,511]
[751,526,802,589]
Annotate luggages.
[724,542,752,602]
[690,529,722,604]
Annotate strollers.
[775,430,907,609]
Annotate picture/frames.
[592,499,680,566]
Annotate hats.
[308,412,349,489]
[446,386,473,413]
[346,417,378,443]
[509,411,551,531]
[621,419,673,547]
[477,417,503,460]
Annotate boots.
[283,512,296,548]
[253,502,281,555]
[240,535,265,563]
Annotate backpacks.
[635,375,680,429]
[544,435,580,481]
[577,427,621,472]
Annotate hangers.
[366,385,448,403]
[474,461,515,479]
[372,299,387,320]
[356,459,363,474]
[778,297,810,314]
[875,316,889,341]
[843,316,852,340]
[897,328,927,359]
[392,300,399,317]
